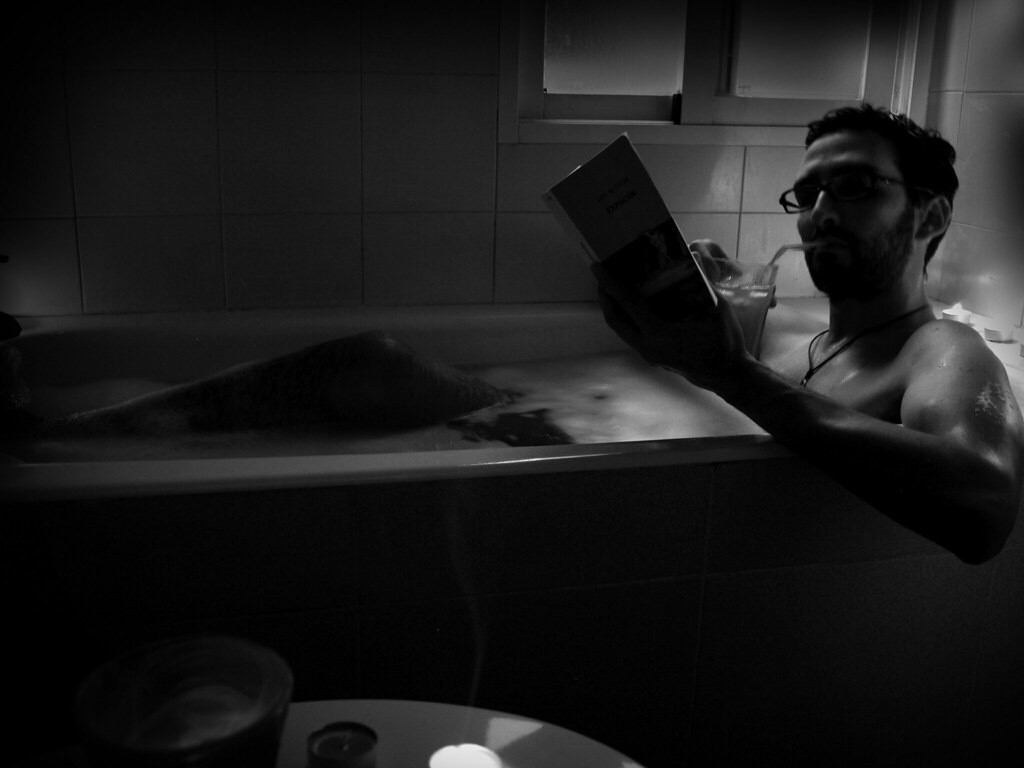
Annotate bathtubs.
[0,289,1024,768]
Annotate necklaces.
[800,307,923,386]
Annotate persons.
[0,106,1024,563]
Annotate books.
[541,136,721,314]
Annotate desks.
[173,699,642,768]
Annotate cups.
[704,254,777,361]
[86,641,297,765]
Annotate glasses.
[779,168,937,214]
